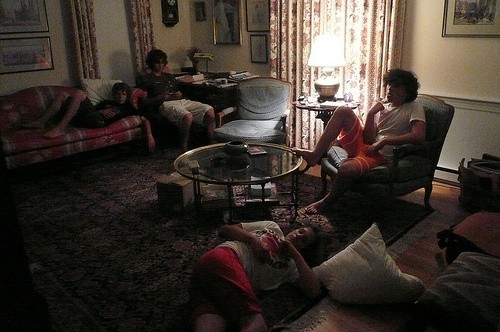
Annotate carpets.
[0,142,436,332]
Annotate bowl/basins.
[313,82,340,98]
[223,140,248,155]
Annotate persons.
[290,69,426,214]
[140,49,219,152]
[20,83,156,155]
[191,220,330,332]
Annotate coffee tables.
[173,142,303,227]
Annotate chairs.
[401,213,500,332]
[321,93,455,220]
[212,76,293,149]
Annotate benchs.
[0,85,147,173]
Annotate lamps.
[193,52,213,72]
[306,34,347,100]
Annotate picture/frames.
[0,35,54,74]
[212,0,243,45]
[0,0,49,34]
[442,0,500,38]
[245,0,270,32]
[250,34,268,63]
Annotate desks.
[292,99,359,190]
[176,72,238,110]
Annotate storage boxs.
[156,166,205,217]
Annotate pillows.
[80,79,123,106]
[0,96,24,133]
[311,221,428,309]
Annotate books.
[250,176,271,197]
[157,174,194,210]
[248,147,267,155]
[202,185,236,209]
[176,72,260,88]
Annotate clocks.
[161,0,179,28]
